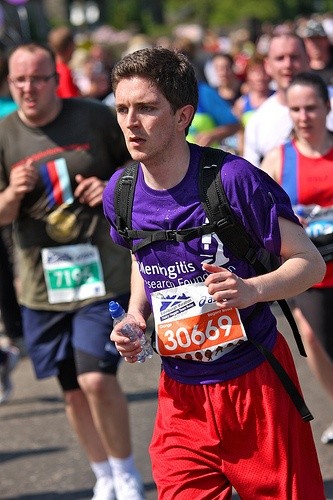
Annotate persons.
[0,55,28,402]
[0,41,146,500]
[233,70,333,446]
[102,46,327,500]
[46,21,333,157]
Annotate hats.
[297,19,327,38]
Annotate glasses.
[9,73,56,87]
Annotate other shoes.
[91,477,116,500]
[113,468,144,500]
[320,425,333,444]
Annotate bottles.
[109,301,153,364]
